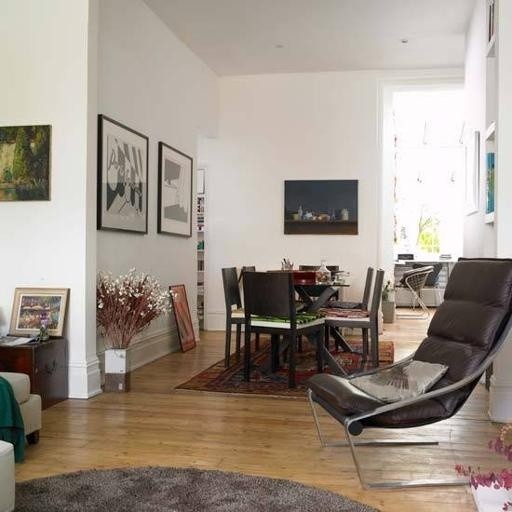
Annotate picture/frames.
[283,179,359,236]
[157,141,194,238]
[0,125,52,203]
[168,283,198,354]
[6,286,71,339]
[96,113,150,236]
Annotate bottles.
[315,259,330,284]
[40,312,49,341]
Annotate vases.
[104,347,132,394]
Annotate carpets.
[171,335,394,402]
[11,462,383,512]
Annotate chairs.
[298,264,341,354]
[317,268,386,373]
[396,265,435,321]
[242,269,326,388]
[304,256,512,490]
[220,266,289,369]
[321,266,374,361]
